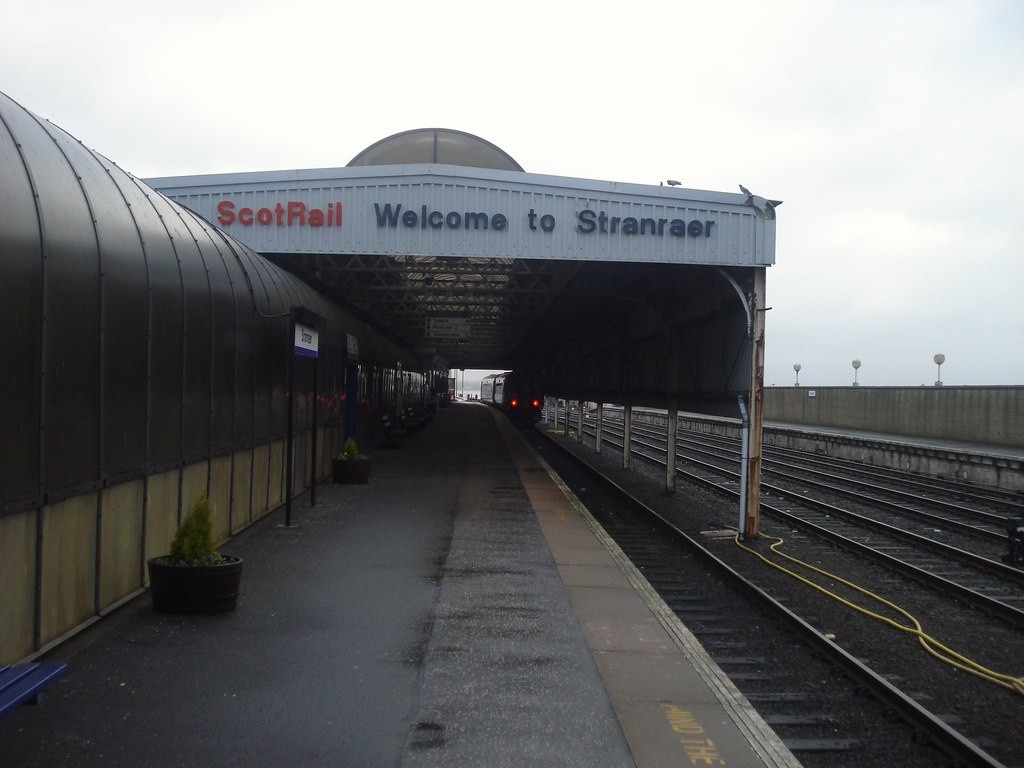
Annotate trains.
[480,371,543,420]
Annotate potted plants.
[149,493,241,613]
[333,437,370,485]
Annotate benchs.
[0,660,72,715]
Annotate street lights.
[792,363,801,387]
[933,353,945,385]
[850,360,861,386]
